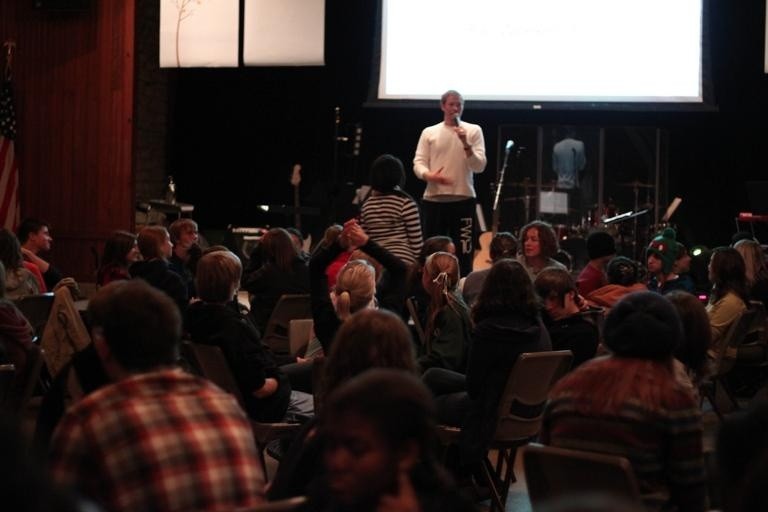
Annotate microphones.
[449,109,469,134]
[504,139,514,151]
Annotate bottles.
[164,175,176,205]
[134,202,151,229]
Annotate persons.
[0,90,767,511]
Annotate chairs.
[12,292,57,418]
[470,349,574,512]
[181,337,302,432]
[522,441,641,512]
[698,310,758,422]
[260,293,311,364]
[737,301,767,380]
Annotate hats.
[642,227,681,278]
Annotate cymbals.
[620,181,655,189]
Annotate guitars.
[290,163,312,253]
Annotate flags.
[0,52,21,234]
